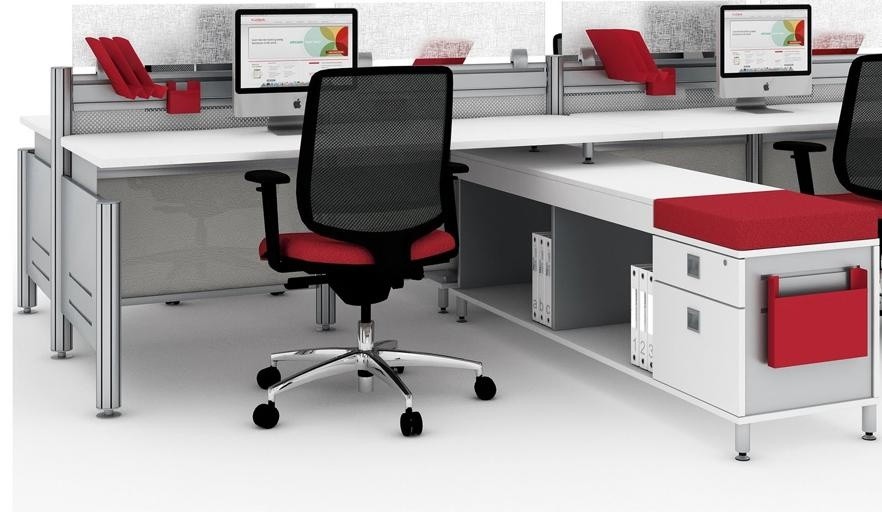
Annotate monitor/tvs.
[231,7,359,136]
[716,3,813,115]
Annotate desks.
[8,102,882,461]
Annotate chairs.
[772,52,882,231]
[250,66,496,437]
